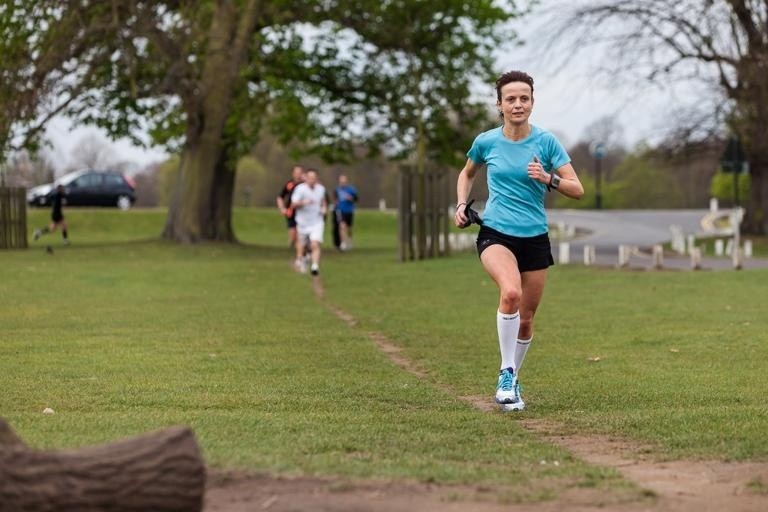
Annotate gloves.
[464,199,483,226]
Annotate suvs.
[27,165,135,211]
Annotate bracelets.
[455,202,466,210]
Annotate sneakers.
[496,368,525,411]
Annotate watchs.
[547,173,560,190]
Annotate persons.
[32,184,70,247]
[453,70,585,411]
[276,163,359,276]
